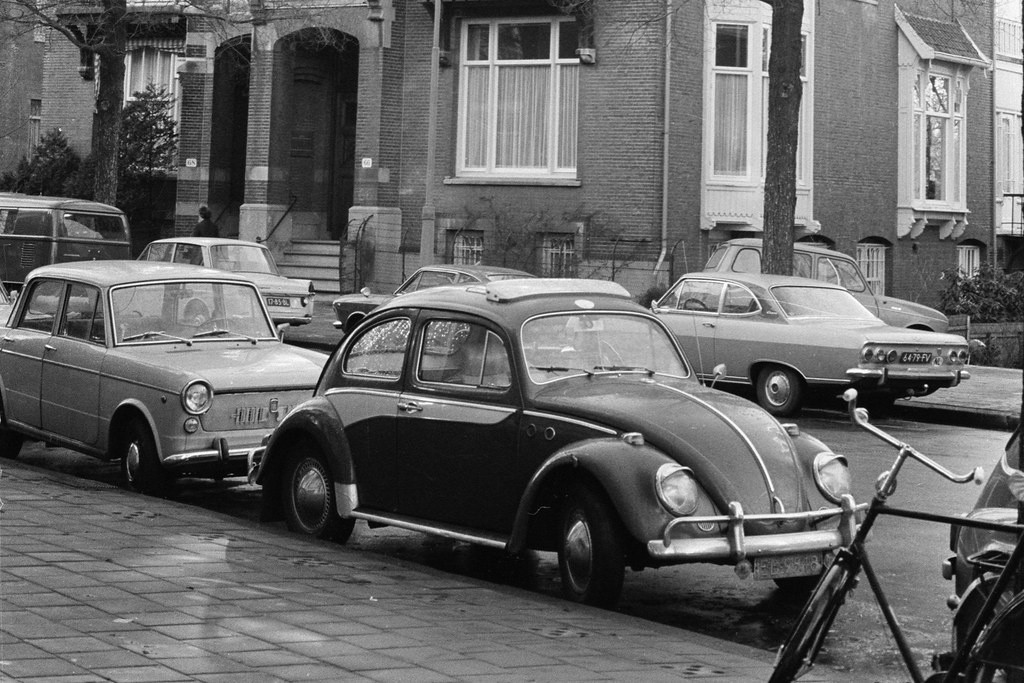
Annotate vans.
[0,192,133,297]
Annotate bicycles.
[766,405,1024,682]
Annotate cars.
[255,276,853,607]
[703,237,948,334]
[137,236,315,332]
[0,260,334,496]
[331,264,536,335]
[648,271,970,416]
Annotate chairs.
[174,296,215,339]
[453,342,510,386]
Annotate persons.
[556,314,611,377]
[190,206,220,267]
